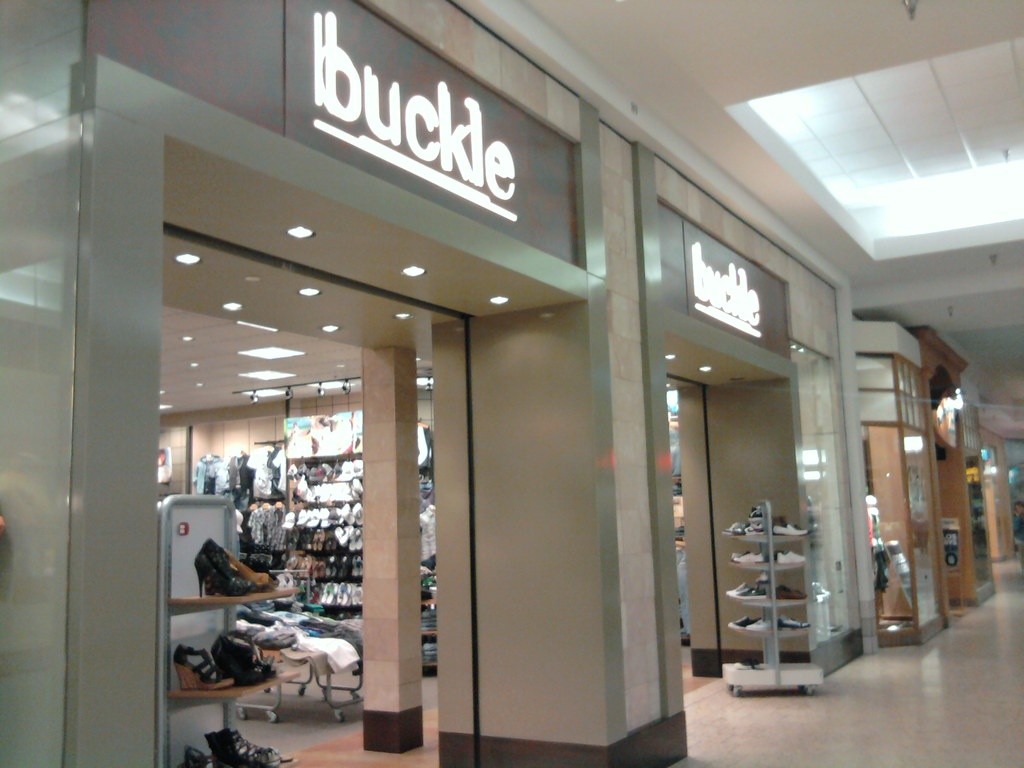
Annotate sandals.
[184,746,221,767]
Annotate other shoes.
[279,458,364,603]
[270,747,292,762]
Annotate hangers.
[195,440,288,517]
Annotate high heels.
[205,728,273,768]
[212,635,263,682]
[195,538,249,597]
[174,641,234,690]
[224,548,270,589]
[227,628,287,674]
[228,730,280,767]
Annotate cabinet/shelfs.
[283,456,362,660]
[159,494,301,768]
[721,499,823,697]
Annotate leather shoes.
[772,523,808,536]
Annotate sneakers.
[777,614,810,631]
[744,619,772,630]
[725,584,749,596]
[734,657,760,670]
[727,616,761,630]
[734,552,764,562]
[734,588,766,598]
[777,586,808,599]
[778,551,807,564]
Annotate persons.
[970,504,989,561]
[866,493,890,594]
[1014,501,1024,582]
